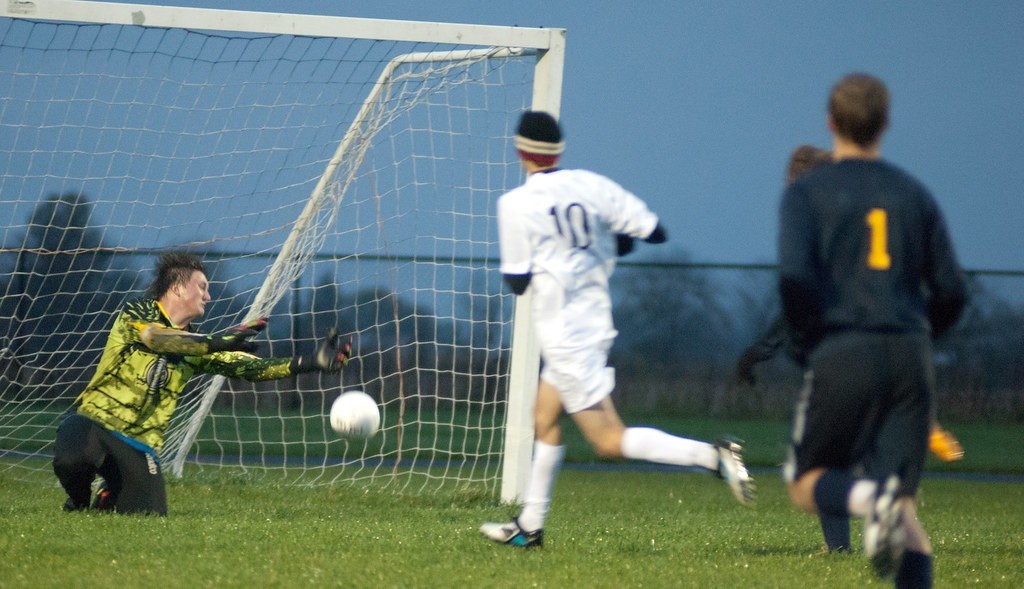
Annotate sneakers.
[863,471,906,583]
[480,517,545,551]
[61,477,116,513]
[712,434,759,510]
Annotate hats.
[513,110,566,164]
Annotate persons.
[52,255,353,517]
[731,143,850,553]
[776,70,970,589]
[479,109,756,549]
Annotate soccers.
[330,390,380,440]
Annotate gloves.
[199,316,270,355]
[292,328,353,375]
